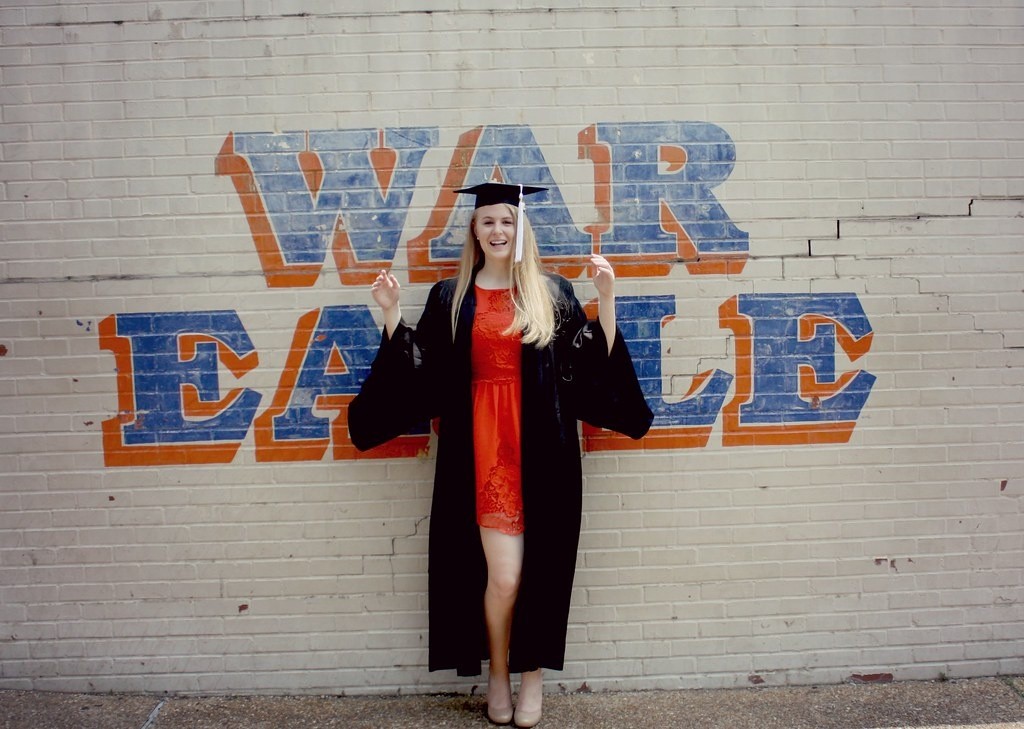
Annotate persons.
[348,183,654,727]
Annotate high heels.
[514,667,543,726]
[488,668,514,723]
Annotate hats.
[453,182,550,264]
